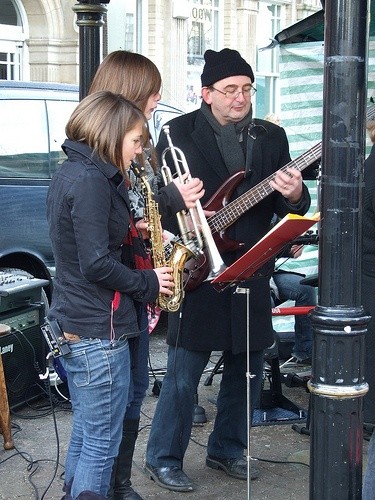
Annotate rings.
[283,183,288,189]
[287,178,292,184]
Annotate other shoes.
[62,477,113,500]
[294,356,312,365]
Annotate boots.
[109,416,144,500]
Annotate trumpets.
[160,123,229,282]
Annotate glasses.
[212,84,258,99]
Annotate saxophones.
[131,160,195,313]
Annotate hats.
[201,48,254,87]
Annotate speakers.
[0,299,48,411]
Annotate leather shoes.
[206,452,259,479]
[146,461,194,492]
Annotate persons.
[260,212,317,363]
[151,48,312,493]
[87,49,206,500]
[45,90,176,500]
[359,93,375,442]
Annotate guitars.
[163,97,374,292]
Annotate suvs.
[0,80,188,308]
